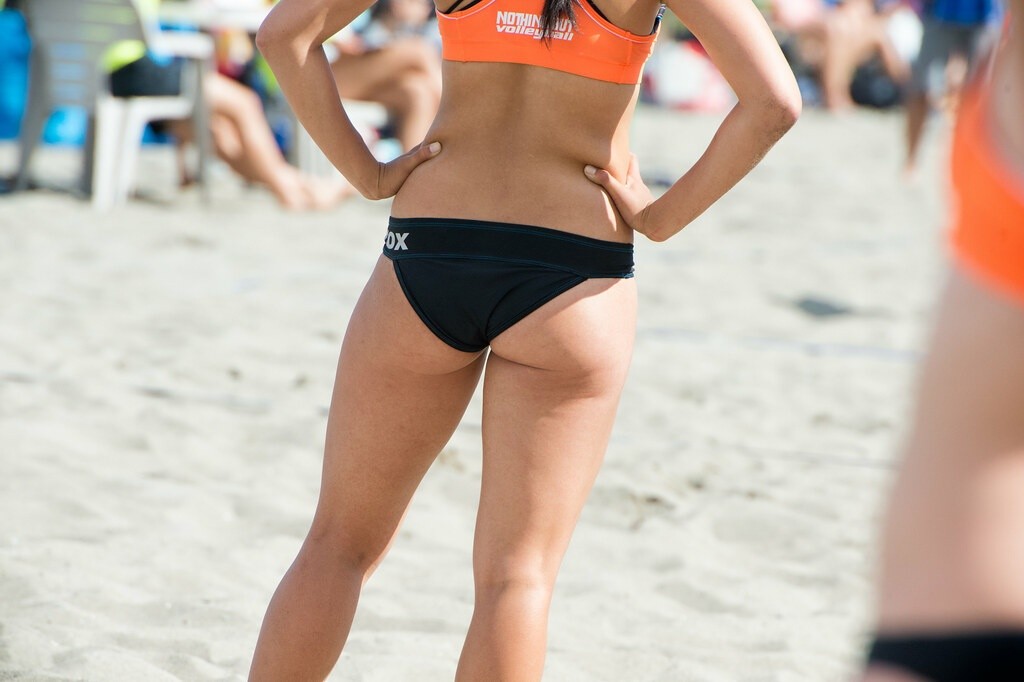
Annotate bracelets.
[946,78,1024,305]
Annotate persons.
[247,0,804,682]
[108,0,442,213]
[640,0,959,119]
[855,0,1024,682]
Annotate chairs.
[16,0,217,205]
